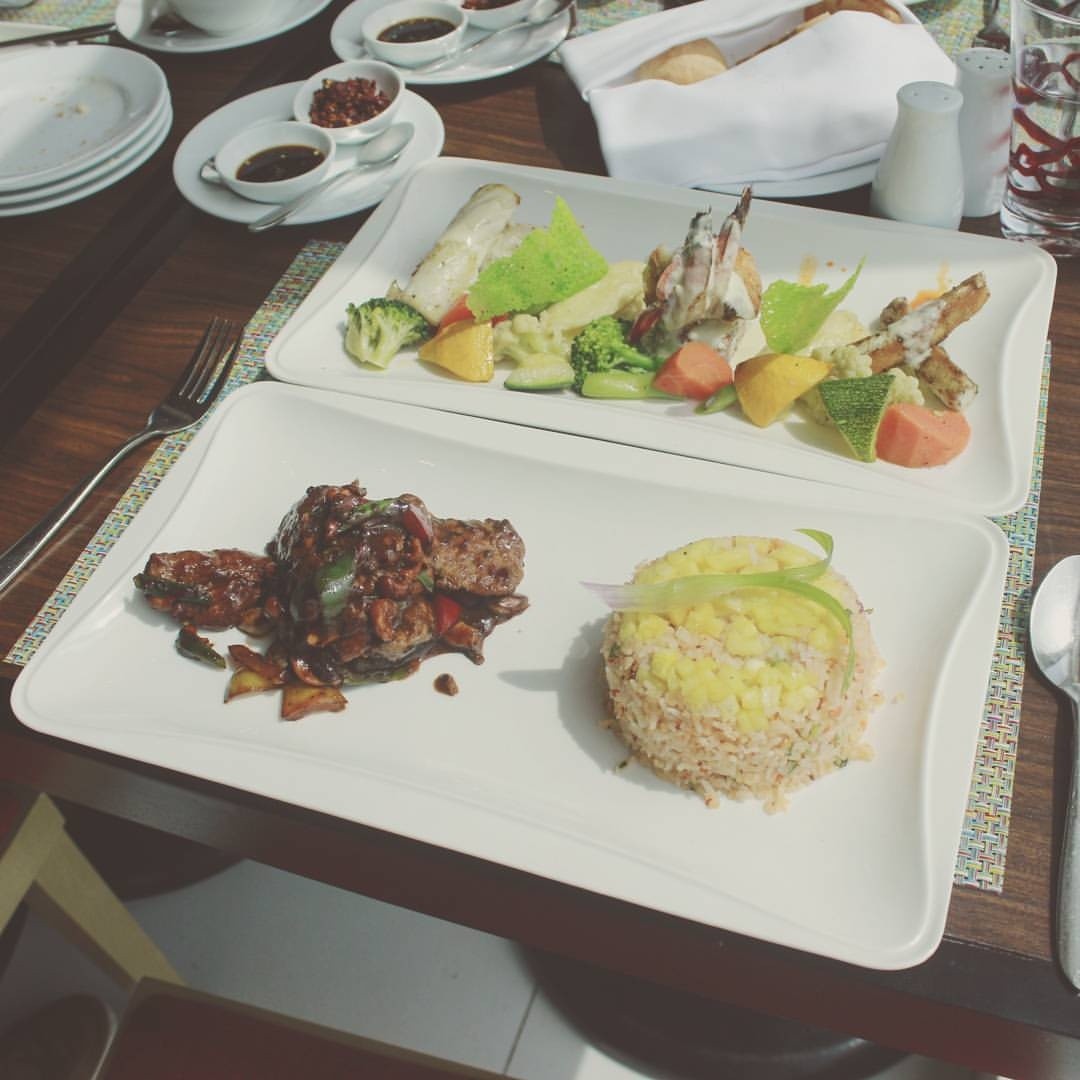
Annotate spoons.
[1030,554,1080,992]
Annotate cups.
[1001,0,1080,258]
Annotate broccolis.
[569,315,662,392]
[344,298,430,366]
[806,344,923,428]
[492,311,562,363]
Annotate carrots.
[652,339,732,398]
[876,404,971,468]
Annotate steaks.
[139,478,529,682]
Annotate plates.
[3,0,1058,972]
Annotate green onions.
[581,528,853,689]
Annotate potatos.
[735,351,830,426]
[419,317,495,382]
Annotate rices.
[604,534,880,800]
